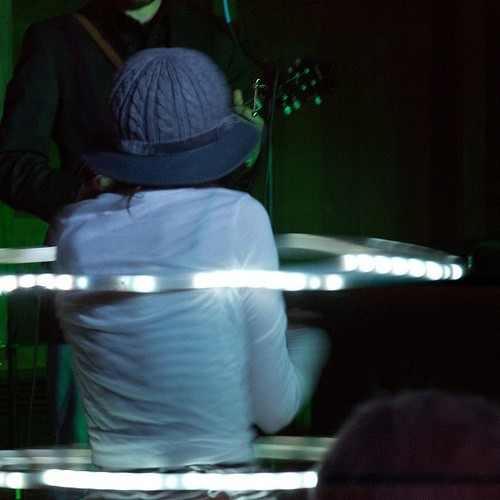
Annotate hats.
[73,47,265,189]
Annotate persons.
[1,1,264,446]
[49,48,329,500]
[317,392,498,499]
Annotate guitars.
[234,45,325,130]
